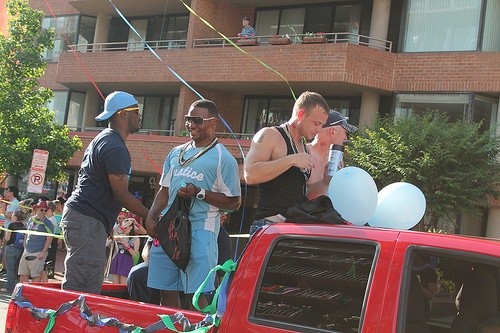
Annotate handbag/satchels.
[155,184,198,274]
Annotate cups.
[327,144,345,176]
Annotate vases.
[269,38,292,44]
[237,39,262,46]
[303,37,327,43]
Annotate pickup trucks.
[5,223,500,333]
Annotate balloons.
[368,182,426,229]
[328,166,378,226]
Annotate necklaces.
[280,124,307,173]
[177,137,219,166]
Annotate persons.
[0,186,49,282]
[0,211,26,292]
[145,100,241,313]
[127,209,234,304]
[18,201,55,281]
[243,92,345,234]
[58,91,149,294]
[237,16,256,36]
[309,110,358,198]
[42,197,66,283]
[105,208,148,286]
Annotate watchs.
[196,188,205,200]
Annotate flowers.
[304,31,326,38]
[238,33,258,39]
[272,33,291,38]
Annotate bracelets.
[120,226,125,231]
[137,224,141,228]
[126,245,130,250]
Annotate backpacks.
[14,229,25,248]
[285,195,346,224]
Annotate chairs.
[451,271,497,333]
[405,277,431,333]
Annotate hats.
[33,200,49,209]
[46,200,56,212]
[322,110,359,133]
[94,90,138,121]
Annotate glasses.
[117,106,140,115]
[220,211,229,217]
[40,209,47,212]
[184,115,216,125]
[329,116,349,125]
